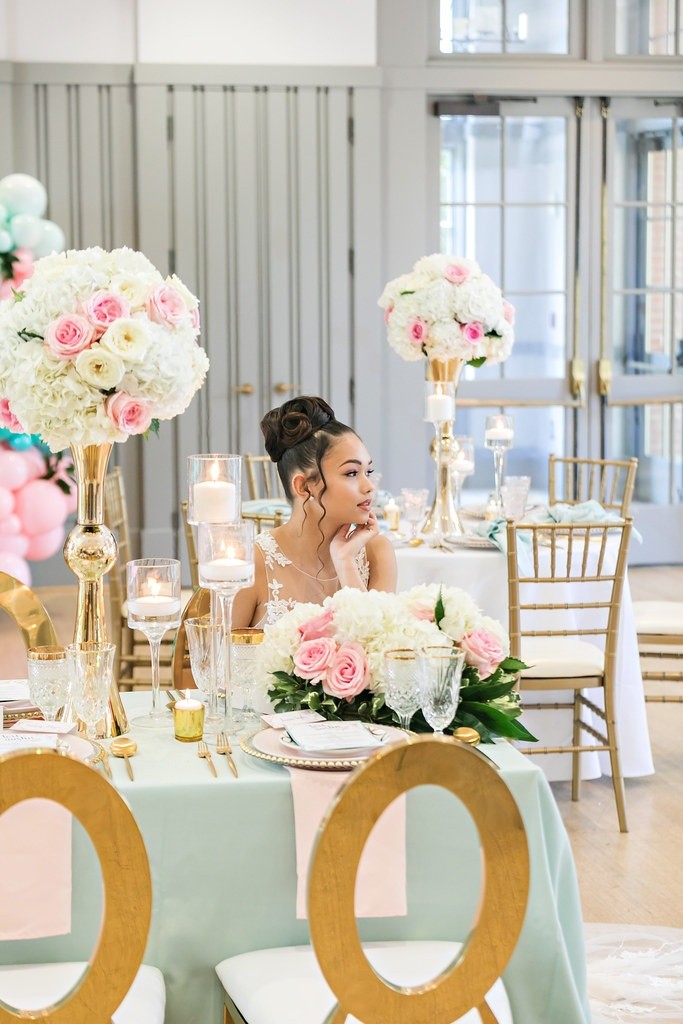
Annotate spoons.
[110,738,137,782]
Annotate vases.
[66,440,138,756]
[419,356,472,547]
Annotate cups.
[172,704,205,743]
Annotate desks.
[2,689,590,1024]
[245,495,655,780]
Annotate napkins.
[283,764,406,920]
[2,798,70,940]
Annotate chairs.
[496,520,636,833]
[215,735,529,1024]
[1,750,166,1024]
[547,457,637,525]
[632,597,683,703]
[0,454,278,686]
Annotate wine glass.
[26,451,264,744]
[382,645,467,734]
[360,380,531,552]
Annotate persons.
[216,395,398,631]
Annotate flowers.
[1,244,209,454]
[265,579,541,749]
[377,253,517,378]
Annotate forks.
[216,733,239,778]
[198,741,218,777]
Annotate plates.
[442,533,497,550]
[238,721,419,771]
